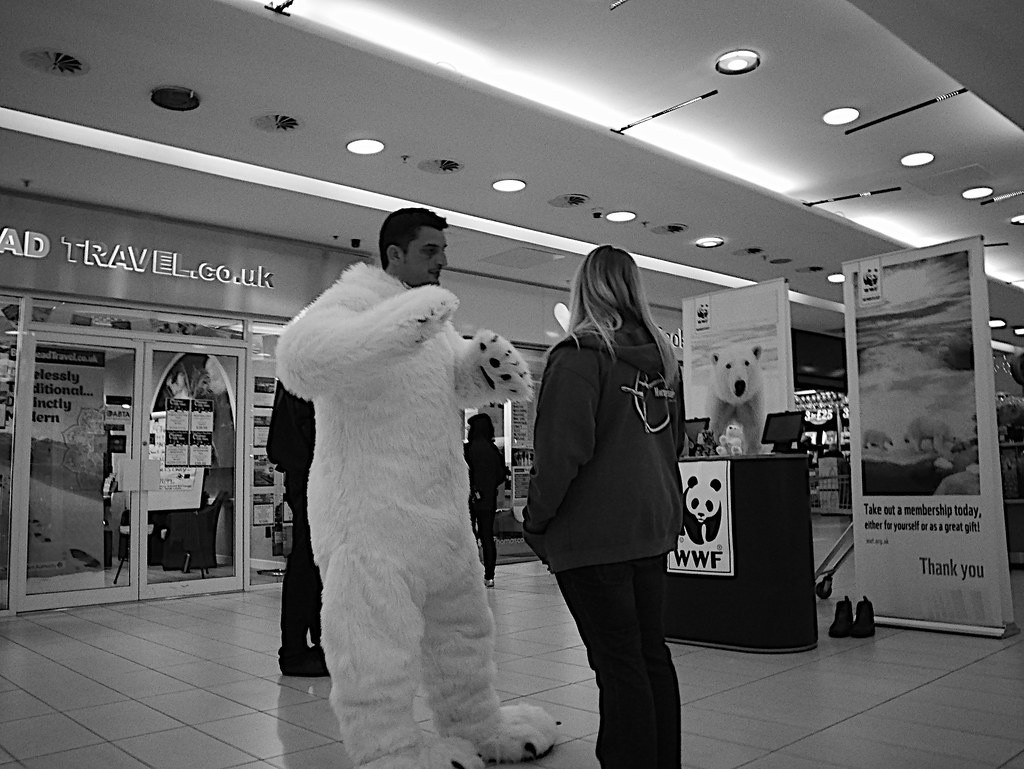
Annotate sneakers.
[485,577,496,590]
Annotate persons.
[266,380,332,677]
[463,412,507,587]
[522,242,686,768]
[275,208,556,767]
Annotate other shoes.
[276,644,329,677]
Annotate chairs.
[162,490,229,575]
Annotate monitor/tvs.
[761,411,804,455]
[684,417,710,456]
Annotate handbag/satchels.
[468,482,487,517]
